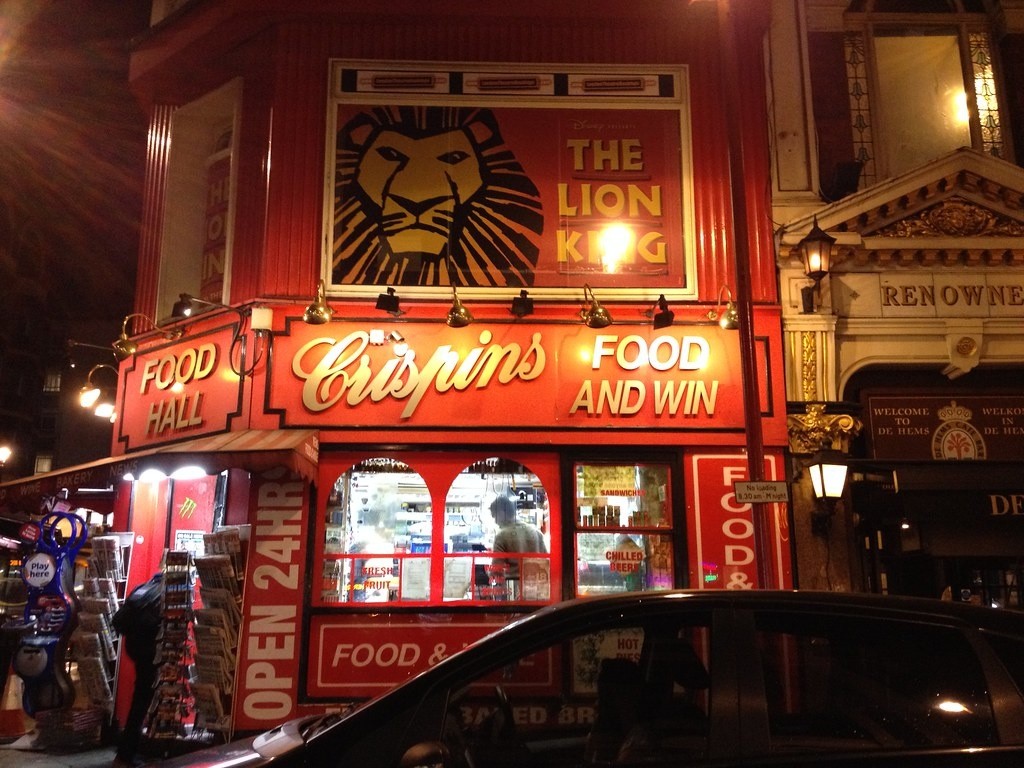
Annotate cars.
[151,591,1024,768]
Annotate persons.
[487,495,549,597]
[111,566,195,768]
[582,657,659,767]
[359,526,395,603]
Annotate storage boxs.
[35,704,107,753]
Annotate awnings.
[0,428,320,517]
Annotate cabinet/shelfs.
[139,526,241,759]
[74,531,135,728]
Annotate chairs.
[613,639,709,761]
[577,656,639,767]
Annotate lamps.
[66,337,130,368]
[303,279,336,326]
[112,313,187,355]
[375,287,402,317]
[804,436,852,537]
[708,285,740,330]
[446,281,474,328]
[34,454,65,474]
[171,293,250,319]
[512,289,534,318]
[647,293,675,331]
[76,364,122,424]
[794,213,838,314]
[578,283,615,329]
